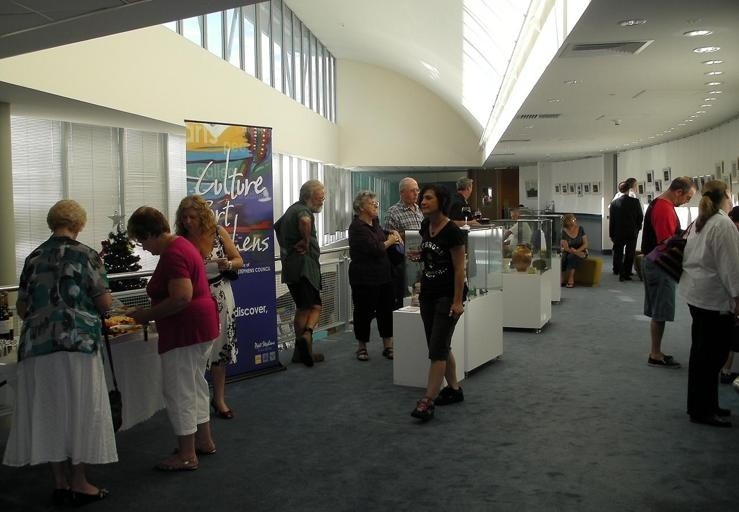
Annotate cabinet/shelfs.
[516,214,567,305]
[391,223,465,389]
[465,226,506,375]
[488,218,553,336]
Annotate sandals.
[196,440,217,455]
[566,282,575,288]
[356,349,370,361]
[157,454,199,470]
[383,348,393,359]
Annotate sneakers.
[435,386,464,405]
[292,334,324,367]
[649,351,682,370]
[411,398,435,421]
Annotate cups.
[0,339,18,367]
[475,211,483,222]
[409,239,421,262]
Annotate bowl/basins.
[148,320,158,334]
[477,218,489,223]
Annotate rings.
[395,236,397,240]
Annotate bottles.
[0,291,14,355]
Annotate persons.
[502,209,532,256]
[124,206,219,471]
[719,350,739,386]
[173,193,243,419]
[383,176,429,302]
[606,177,640,276]
[560,214,589,287]
[0,198,119,507]
[445,176,479,227]
[273,178,329,367]
[640,176,693,368]
[346,190,405,360]
[683,177,738,426]
[609,182,645,281]
[405,184,467,419]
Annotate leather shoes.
[614,269,634,281]
[55,484,110,504]
[688,406,733,428]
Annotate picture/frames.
[553,181,601,199]
[636,159,739,210]
[524,178,538,200]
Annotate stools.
[562,255,603,288]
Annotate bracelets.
[226,260,233,271]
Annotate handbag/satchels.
[646,234,686,283]
[218,256,239,280]
[387,232,405,266]
[109,390,122,433]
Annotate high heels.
[211,398,234,419]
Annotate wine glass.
[462,206,471,227]
